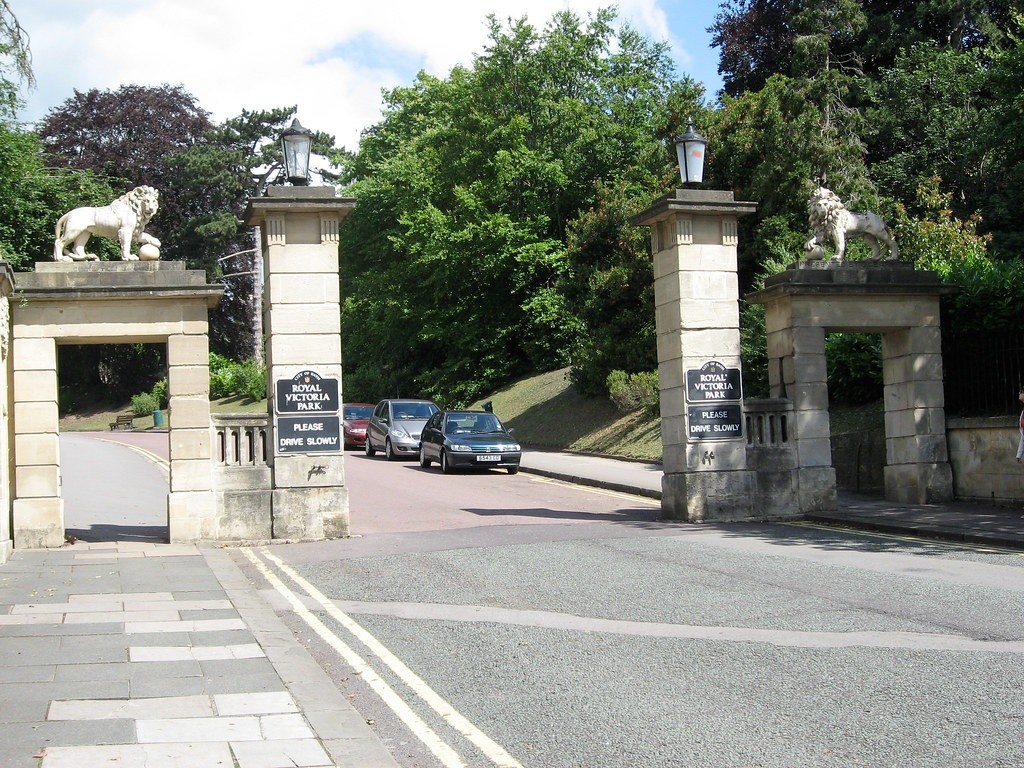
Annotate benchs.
[109,416,134,431]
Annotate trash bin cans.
[153,411,164,427]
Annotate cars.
[419,410,522,474]
[343,403,376,450]
[365,399,441,460]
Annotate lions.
[803,187,898,261]
[54,185,159,263]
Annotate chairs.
[473,422,485,431]
[447,421,458,433]
